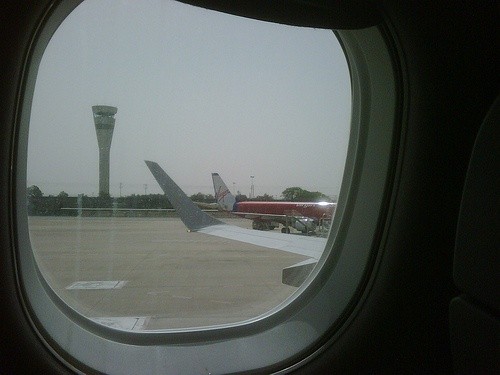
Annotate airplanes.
[192,171,338,235]
